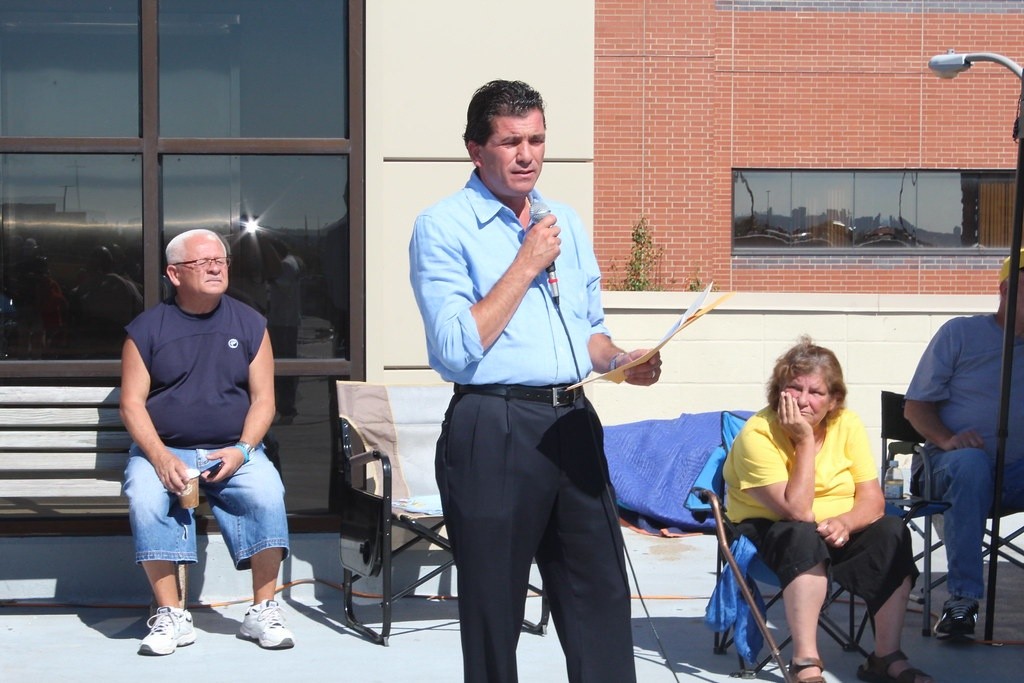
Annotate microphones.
[530,202,560,304]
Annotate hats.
[998,248,1024,282]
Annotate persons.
[119,229,295,657]
[904,250,1024,641]
[0,175,350,359]
[409,79,662,683]
[722,341,934,683]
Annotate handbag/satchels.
[391,495,443,516]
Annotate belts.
[454,382,583,408]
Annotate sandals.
[857,651,932,683]
[789,657,826,683]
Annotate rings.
[652,371,656,379]
[839,538,845,547]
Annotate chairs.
[335,380,549,646]
[879,391,1024,637]
[682,411,952,683]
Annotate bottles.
[884,460,903,510]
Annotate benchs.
[0,386,206,621]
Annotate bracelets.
[611,352,625,370]
[236,445,248,464]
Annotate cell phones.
[207,462,224,478]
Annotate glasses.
[173,257,231,269]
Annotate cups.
[175,468,201,509]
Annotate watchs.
[238,440,256,461]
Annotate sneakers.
[933,597,979,640]
[240,599,295,648]
[136,606,196,655]
